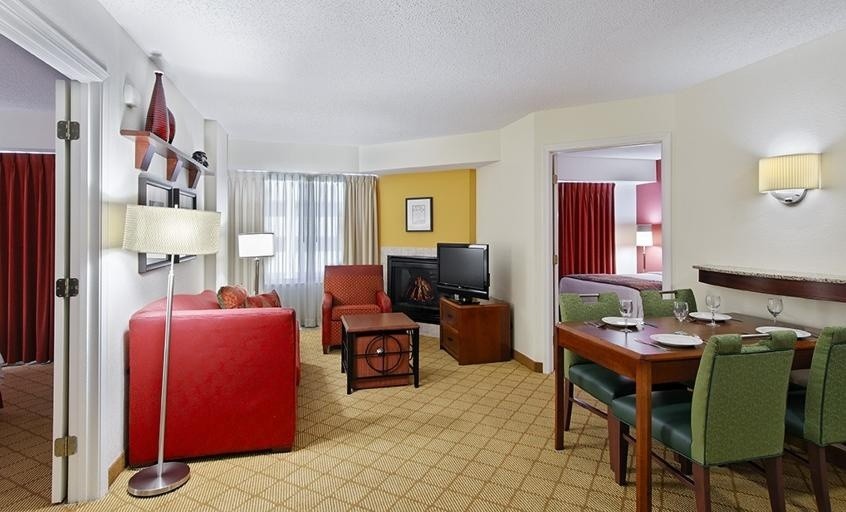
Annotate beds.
[558,272,662,316]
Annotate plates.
[688,311,732,322]
[601,315,638,328]
[755,325,811,340]
[648,333,705,347]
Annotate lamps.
[634,222,654,273]
[757,152,822,207]
[120,201,225,501]
[237,232,277,295]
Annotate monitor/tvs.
[437,243,489,306]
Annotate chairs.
[560,293,689,471]
[639,288,698,315]
[785,325,844,510]
[608,331,797,512]
[319,263,394,355]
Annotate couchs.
[126,288,302,469]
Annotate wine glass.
[618,299,634,333]
[704,293,722,328]
[767,297,784,326]
[672,300,690,336]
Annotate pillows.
[247,288,282,308]
[216,282,250,309]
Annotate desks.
[554,312,823,485]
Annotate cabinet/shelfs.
[438,296,511,366]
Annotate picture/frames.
[172,186,199,263]
[403,195,435,233]
[137,172,175,274]
